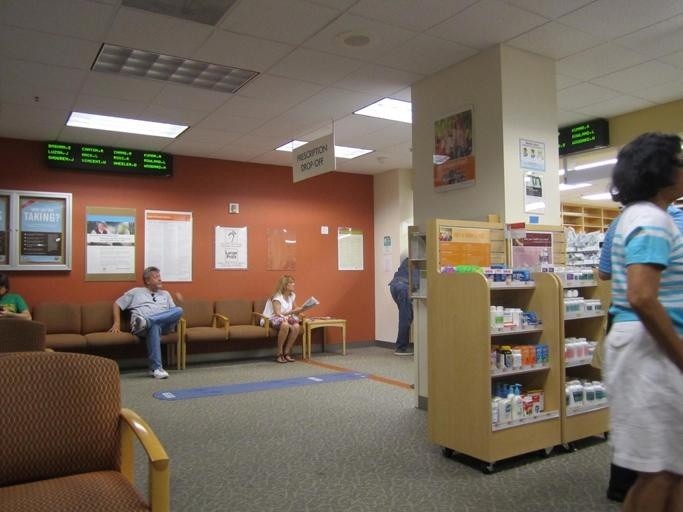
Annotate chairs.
[0,317,54,352]
[0,352,171,512]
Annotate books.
[303,296,320,311]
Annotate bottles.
[489,303,549,373]
[541,261,608,409]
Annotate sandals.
[273,351,295,362]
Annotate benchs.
[31,302,181,370]
[171,298,306,371]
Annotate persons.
[387,257,414,355]
[596,206,683,501]
[603,131,683,512]
[533,177,542,188]
[257,275,306,364]
[0,273,53,351]
[438,118,471,160]
[105,267,184,379]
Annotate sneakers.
[394,344,414,355]
[149,366,169,379]
[607,488,625,500]
[131,316,147,334]
